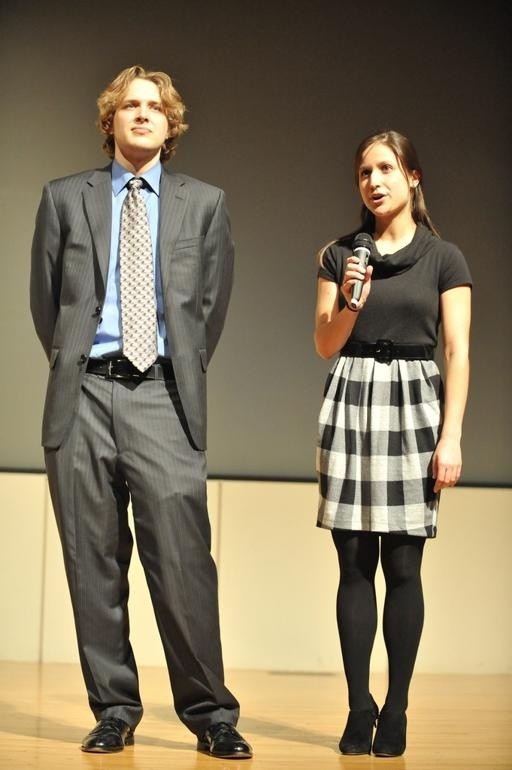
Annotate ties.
[118,179,159,375]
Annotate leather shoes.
[373,704,406,755]
[81,717,135,753]
[339,693,379,755]
[197,721,253,759]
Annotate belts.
[86,357,174,381]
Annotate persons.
[27,64,258,761]
[309,125,475,759]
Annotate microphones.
[350,232,374,309]
